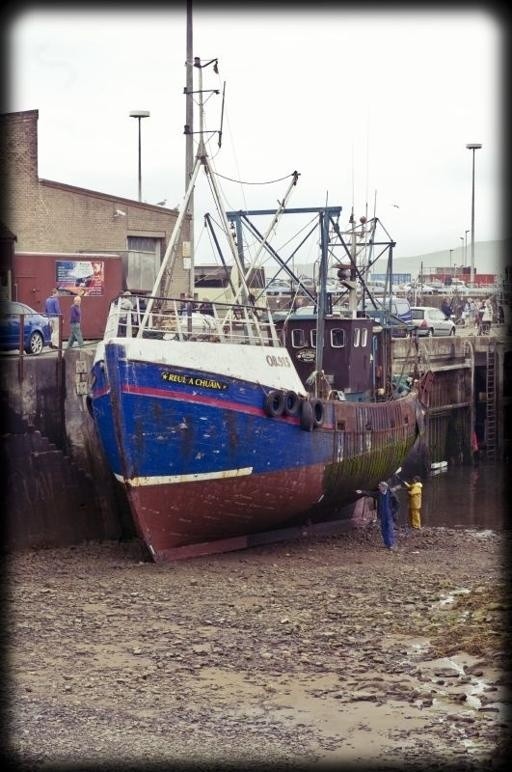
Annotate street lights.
[463,140,484,288]
[449,230,470,268]
[127,109,151,205]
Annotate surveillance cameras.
[116,210,125,216]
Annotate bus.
[190,259,268,329]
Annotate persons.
[473,297,493,336]
[114,285,215,340]
[392,469,424,532]
[441,296,454,319]
[66,294,88,348]
[44,287,65,350]
[355,480,401,551]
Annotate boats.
[82,1,436,573]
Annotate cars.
[254,273,507,340]
[0,295,53,355]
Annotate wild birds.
[156,197,167,207]
